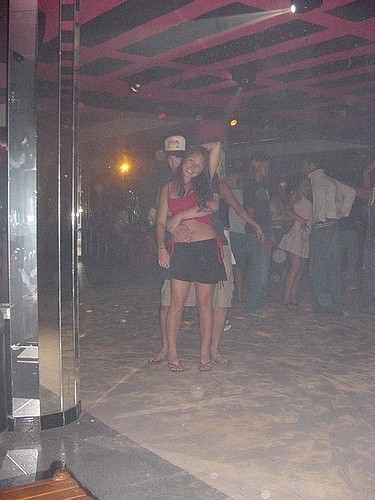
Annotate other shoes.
[200,360,212,371]
[280,295,304,310]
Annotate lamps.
[130,81,142,93]
[291,0,323,14]
[232,63,256,86]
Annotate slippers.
[214,354,234,367]
[168,361,184,371]
[146,352,166,365]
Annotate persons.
[85,135,375,333]
[147,135,234,367]
[156,141,228,373]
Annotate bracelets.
[157,246,166,250]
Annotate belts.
[313,221,339,230]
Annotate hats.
[164,135,185,158]
